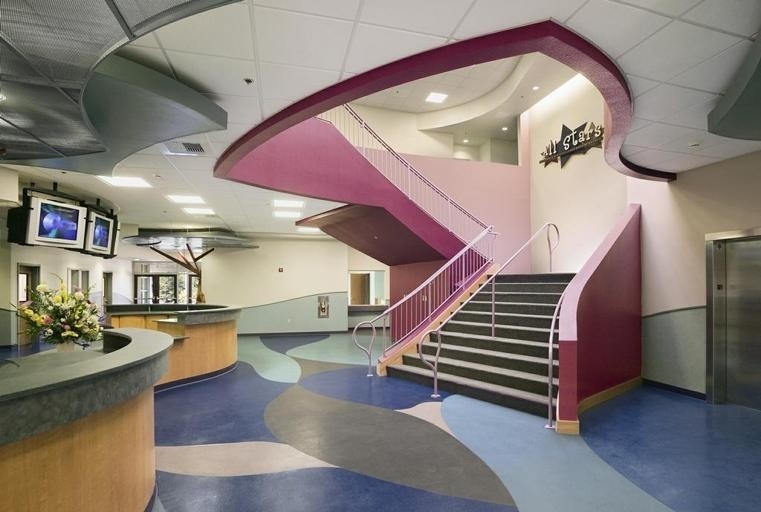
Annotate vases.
[55,340,73,352]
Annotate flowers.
[8,272,109,352]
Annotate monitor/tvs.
[64,210,114,256]
[6,196,88,250]
[103,220,121,259]
